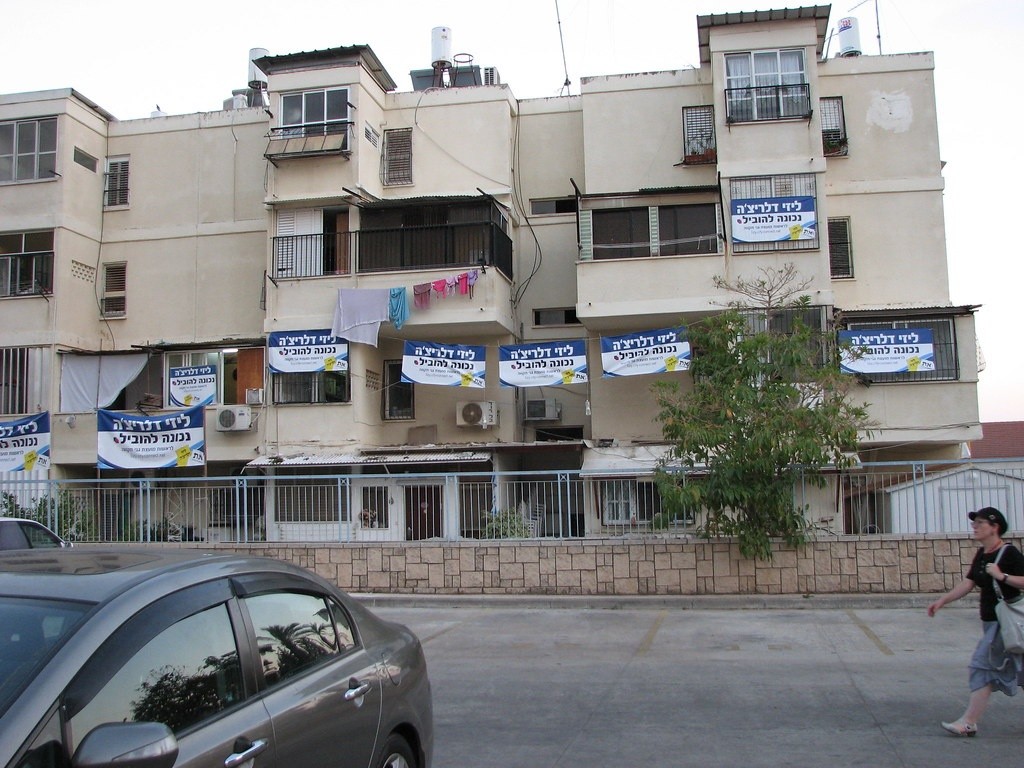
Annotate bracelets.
[1001,574,1008,582]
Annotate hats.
[968,507,1007,535]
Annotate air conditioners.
[223,95,250,110]
[525,398,559,421]
[456,401,499,426]
[485,67,500,85]
[215,406,253,431]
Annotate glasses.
[971,520,991,527]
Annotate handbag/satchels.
[994,598,1024,655]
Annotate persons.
[928,506,1024,738]
[408,497,437,541]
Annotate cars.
[0,546,437,768]
[0,517,74,551]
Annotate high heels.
[940,718,977,737]
